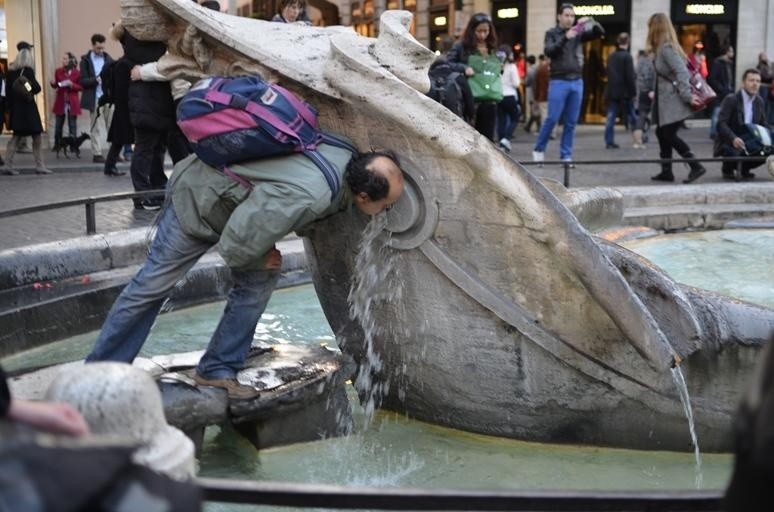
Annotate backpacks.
[101,60,131,103]
[176,75,358,202]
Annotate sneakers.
[135,199,162,210]
[500,137,510,149]
[606,144,619,149]
[559,158,574,168]
[532,151,544,165]
[192,376,259,400]
[722,166,754,178]
[634,142,645,146]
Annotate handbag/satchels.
[466,55,504,101]
[12,76,35,103]
[737,123,773,156]
[676,71,717,112]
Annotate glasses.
[475,15,492,21]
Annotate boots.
[3,140,19,175]
[32,135,52,173]
[655,149,705,184]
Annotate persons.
[0,365,89,441]
[81,137,406,401]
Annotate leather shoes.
[104,168,126,175]
[52,145,131,163]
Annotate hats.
[17,42,34,50]
